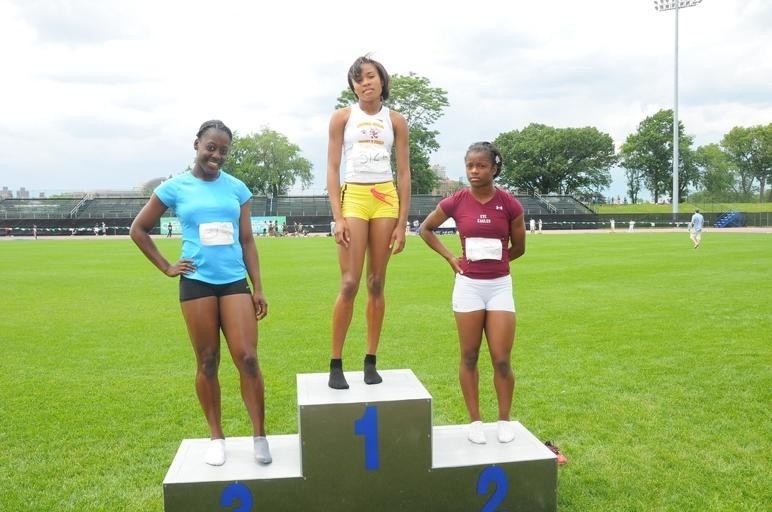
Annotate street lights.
[652,0,704,212]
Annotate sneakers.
[544,440,566,464]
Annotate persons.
[417,140,528,445]
[536,218,543,234]
[129,119,272,466]
[405,219,410,232]
[628,218,636,233]
[325,54,411,390]
[102,221,108,237]
[263,220,304,235]
[166,222,173,238]
[328,219,337,236]
[529,217,536,235]
[609,217,615,231]
[413,217,420,233]
[688,208,705,249]
[94,223,100,237]
